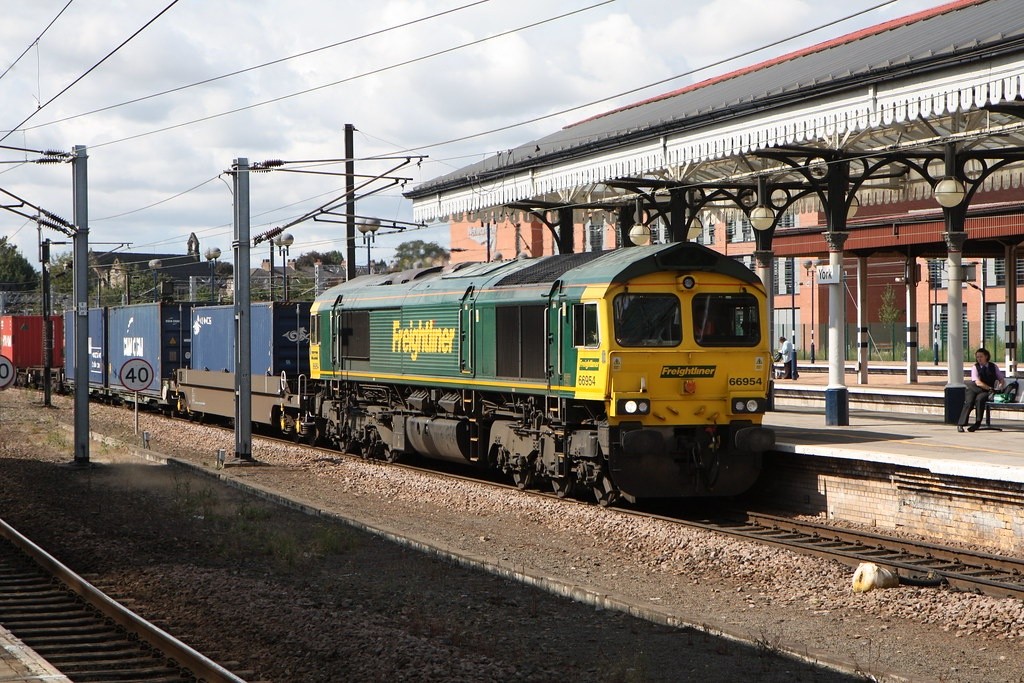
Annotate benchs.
[977,378,1024,431]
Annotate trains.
[0,242,777,508]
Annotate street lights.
[148,259,162,301]
[358,217,381,274]
[274,234,294,302]
[203,247,222,301]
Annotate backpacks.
[1002,384,1019,401]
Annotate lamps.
[627,197,652,245]
[747,173,775,230]
[932,140,965,208]
[685,191,703,239]
[844,192,859,219]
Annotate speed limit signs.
[119,358,155,392]
[0,355,16,391]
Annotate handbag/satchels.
[987,389,1011,403]
[774,353,781,361]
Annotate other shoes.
[968,424,979,432]
[957,425,964,432]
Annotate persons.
[957,349,1004,433]
[695,306,717,337]
[777,337,799,379]
